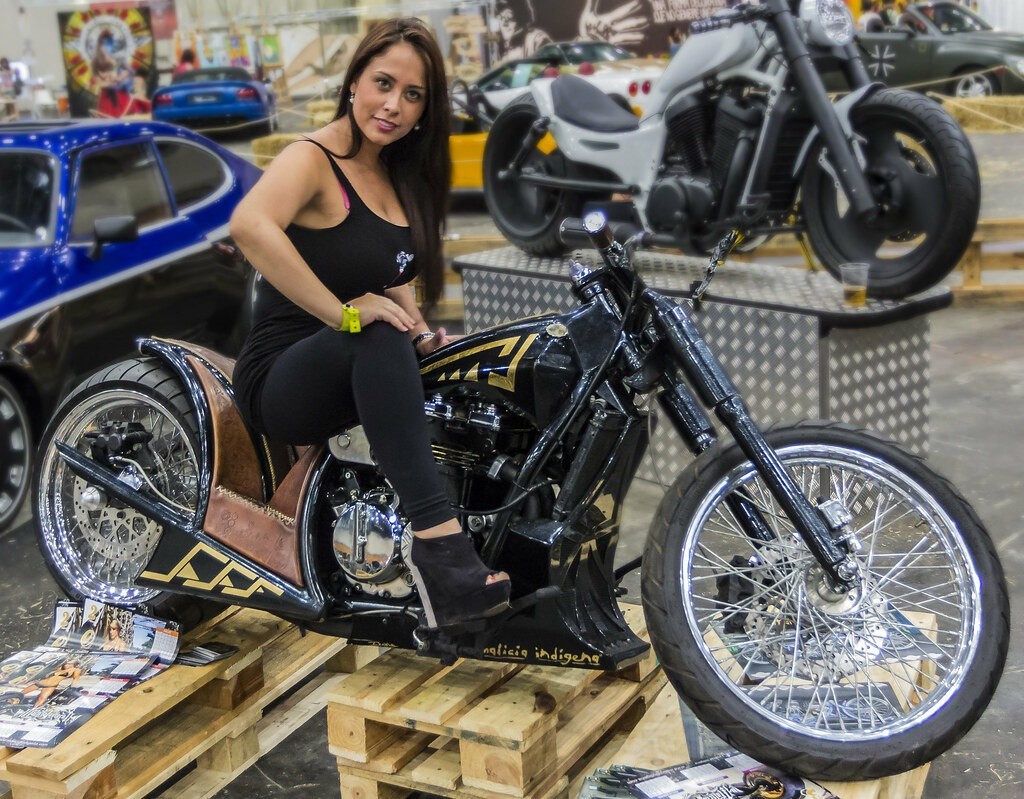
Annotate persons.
[230,17,513,620]
[18,658,81,708]
[173,49,194,83]
[0,58,17,115]
[99,620,125,654]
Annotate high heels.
[401,521,511,629]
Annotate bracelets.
[412,332,435,348]
[333,304,361,333]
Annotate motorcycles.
[465,0,982,300]
[23,208,1013,782]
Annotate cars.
[433,32,670,125]
[0,114,267,538]
[149,56,282,142]
[850,1,1024,95]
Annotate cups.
[839,262,870,307]
